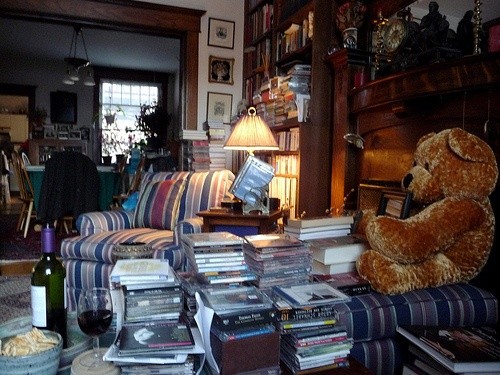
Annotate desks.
[22,165,120,214]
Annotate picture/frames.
[208,17,236,48]
[207,92,233,126]
[208,54,235,85]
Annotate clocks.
[382,20,419,57]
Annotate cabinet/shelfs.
[197,205,284,236]
[241,0,326,226]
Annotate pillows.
[128,179,189,229]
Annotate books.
[244,59,312,128]
[243,127,299,225]
[358,180,412,219]
[178,120,226,173]
[284,215,372,278]
[395,323,500,375]
[175,230,355,375]
[276,11,314,62]
[247,0,261,12]
[102,258,206,375]
[244,39,271,76]
[249,3,273,41]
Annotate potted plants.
[98,102,130,163]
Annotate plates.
[1,311,95,375]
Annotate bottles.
[31,222,69,348]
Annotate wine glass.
[77,288,114,368]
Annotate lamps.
[224,107,280,158]
[61,28,96,88]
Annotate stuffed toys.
[356,127,499,296]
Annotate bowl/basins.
[0,329,63,375]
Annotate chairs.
[0,147,144,237]
[377,189,418,218]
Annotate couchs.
[319,269,500,375]
[60,168,235,315]
